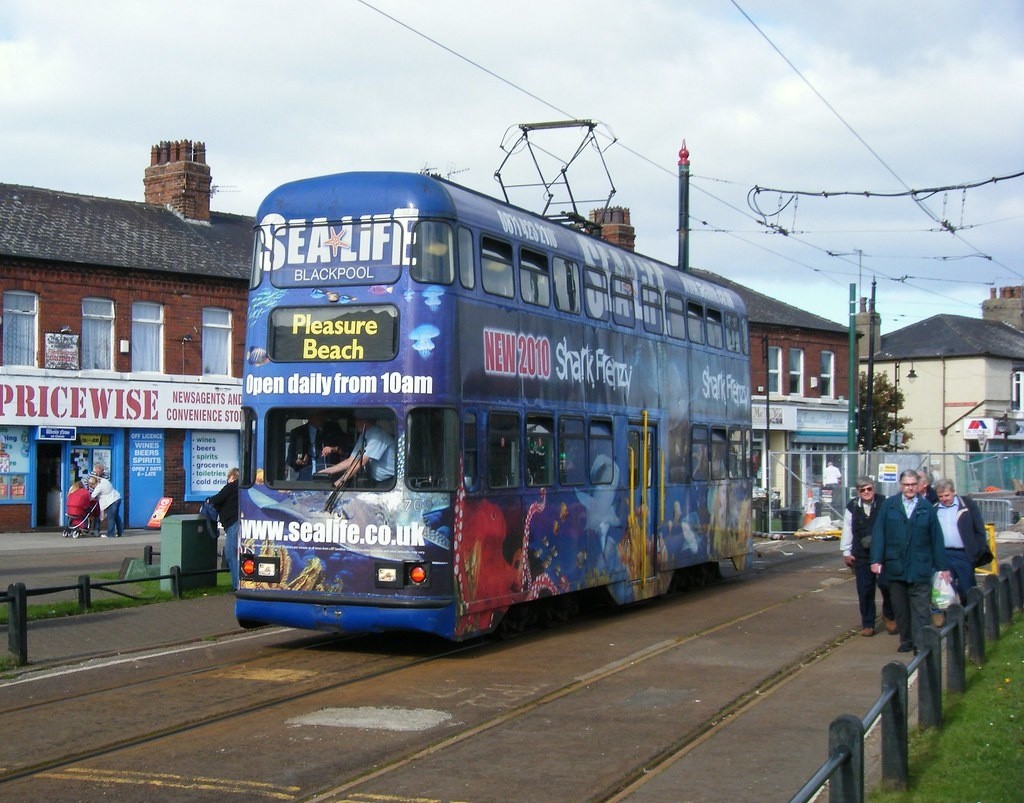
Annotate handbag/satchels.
[931,571,956,610]
[198,501,220,539]
[972,543,994,568]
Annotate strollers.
[62,488,99,539]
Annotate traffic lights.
[996,422,1008,433]
[1008,420,1020,434]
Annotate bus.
[233,171,756,642]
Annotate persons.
[84,463,109,537]
[839,470,994,656]
[824,462,841,508]
[207,468,239,591]
[286,411,397,490]
[88,475,123,538]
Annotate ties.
[315,427,325,473]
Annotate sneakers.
[880,610,898,634]
[862,628,874,637]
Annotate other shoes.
[101,534,107,537]
[92,529,101,537]
[898,644,911,652]
[114,534,118,537]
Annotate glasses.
[858,487,873,493]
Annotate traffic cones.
[803,491,816,527]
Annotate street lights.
[894,359,918,452]
